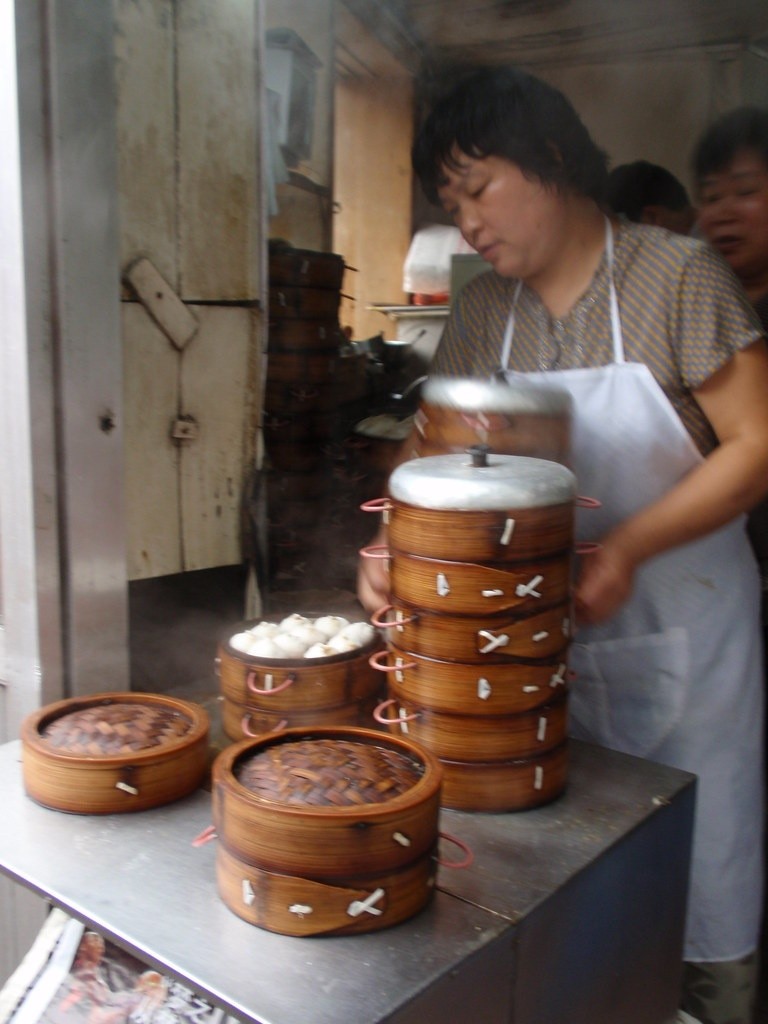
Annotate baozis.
[228,613,377,658]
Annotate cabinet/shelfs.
[0,673,699,1024]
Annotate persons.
[596,108,768,618]
[356,73,768,1024]
